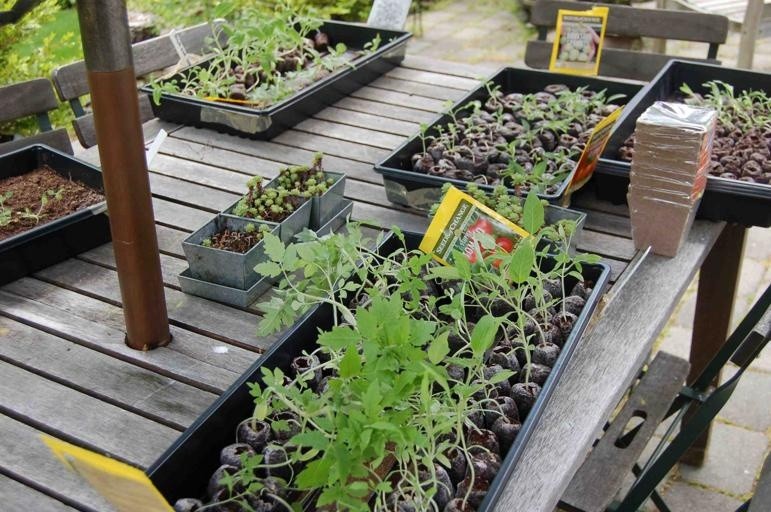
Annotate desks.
[1,57,734,512]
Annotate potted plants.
[373,65,648,214]
[0,141,111,303]
[596,57,770,231]
[176,150,353,306]
[143,227,610,512]
[138,14,415,141]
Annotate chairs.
[51,18,235,149]
[556,278,771,511]
[652,0,769,72]
[0,77,73,158]
[525,0,730,84]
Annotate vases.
[622,101,716,258]
